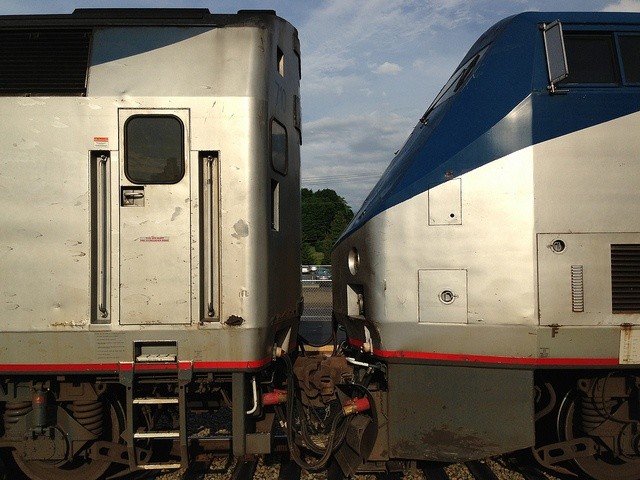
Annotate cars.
[313,268,332,287]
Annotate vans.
[302,265,311,273]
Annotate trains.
[1,8,640,480]
